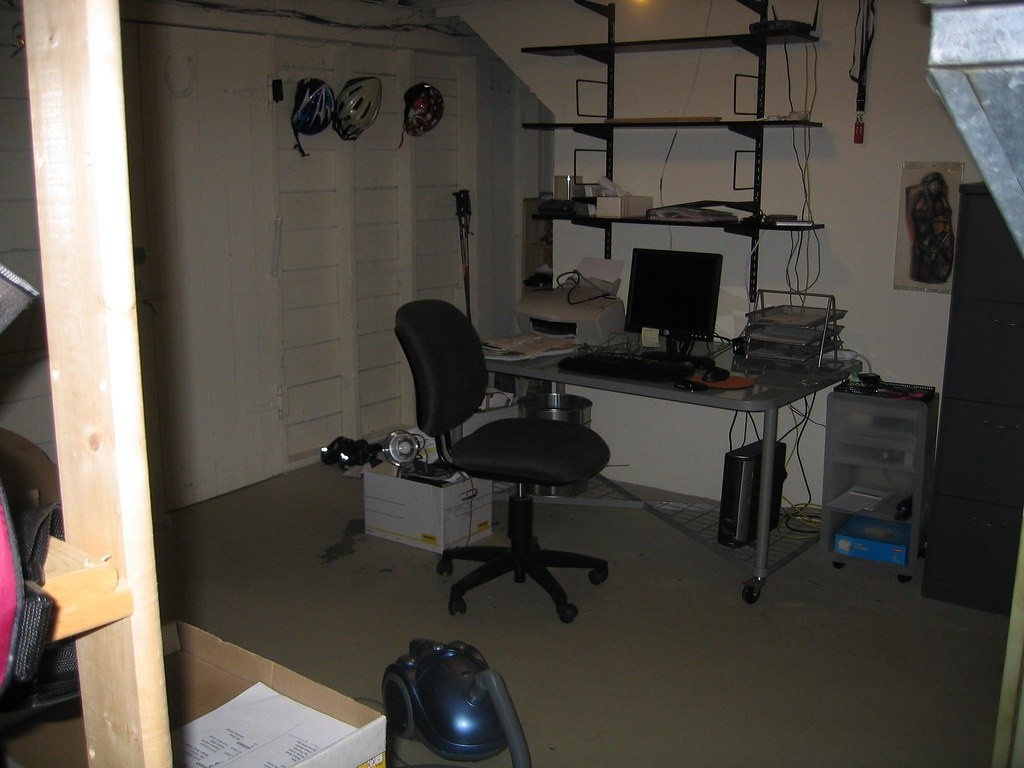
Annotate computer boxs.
[717,439,787,548]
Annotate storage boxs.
[835,517,909,566]
[554,176,583,199]
[0,619,386,768]
[364,463,490,556]
[595,195,653,219]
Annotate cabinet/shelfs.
[821,391,937,583]
[521,33,824,238]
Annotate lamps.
[382,430,426,466]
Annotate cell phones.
[673,368,696,391]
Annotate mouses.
[702,368,731,382]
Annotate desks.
[479,336,865,605]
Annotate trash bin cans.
[514,391,596,496]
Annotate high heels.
[321,436,354,465]
[337,439,384,471]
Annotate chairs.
[395,301,611,624]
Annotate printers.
[515,276,628,347]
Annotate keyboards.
[558,350,694,384]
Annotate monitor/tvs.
[624,248,724,366]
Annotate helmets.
[291,78,335,136]
[332,77,383,141]
[404,81,445,136]
